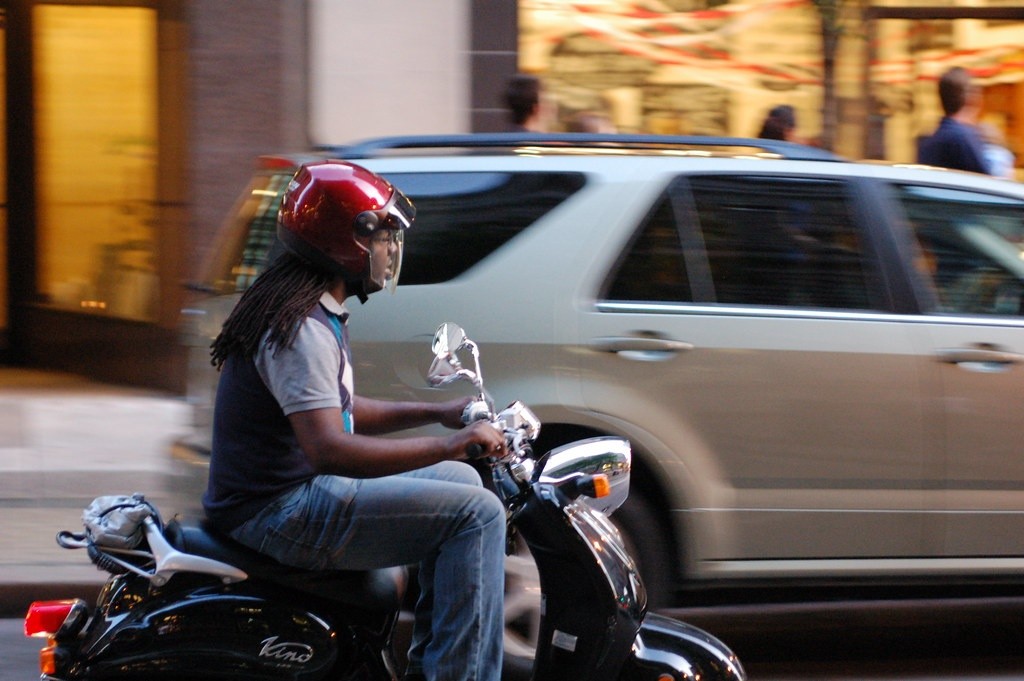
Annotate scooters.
[21,319,753,681]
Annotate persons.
[924,67,994,283]
[201,161,507,681]
[744,104,814,261]
[503,79,619,146]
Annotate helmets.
[277,159,415,305]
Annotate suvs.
[169,130,1024,642]
